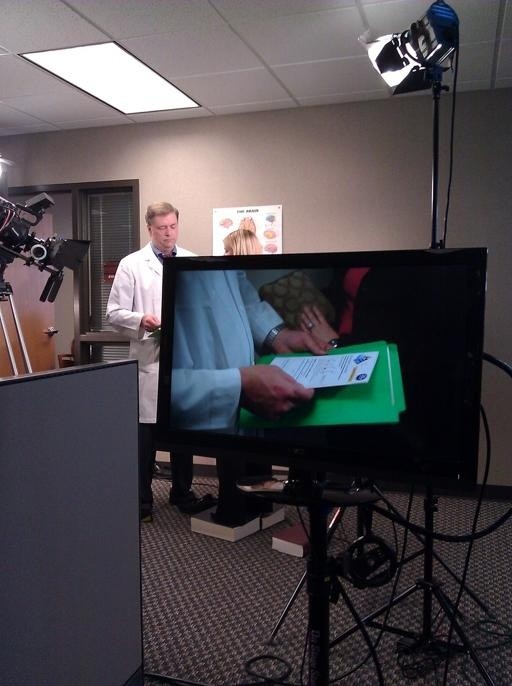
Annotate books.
[238,338,407,429]
[272,522,310,559]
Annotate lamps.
[358,0,459,654]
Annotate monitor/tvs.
[155,247,489,499]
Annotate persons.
[105,202,205,520]
[168,266,334,433]
[299,263,452,435]
[222,229,263,255]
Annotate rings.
[306,321,314,329]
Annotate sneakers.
[140,497,153,521]
[169,489,199,514]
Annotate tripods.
[268,477,511,644]
[265,500,387,684]
[332,72,511,686]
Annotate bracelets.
[263,323,288,349]
